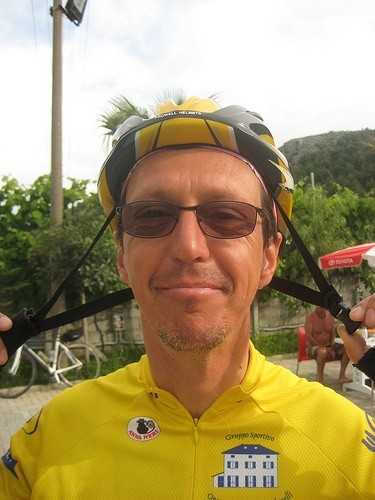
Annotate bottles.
[362,326,368,341]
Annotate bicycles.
[0,325,100,399]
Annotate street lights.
[49,1,88,317]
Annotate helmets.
[95,98,295,270]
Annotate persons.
[1,95,375,500]
[303,300,354,387]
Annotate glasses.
[117,200,271,239]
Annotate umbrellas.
[318,243,375,270]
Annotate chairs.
[296,328,341,382]
